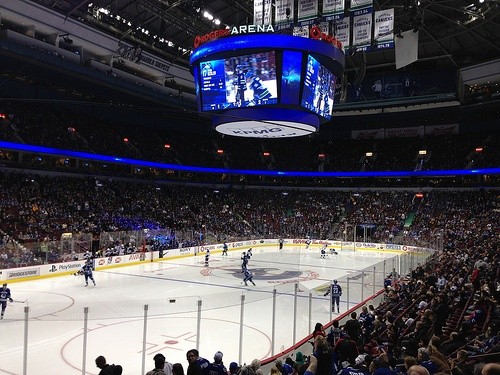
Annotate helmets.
[236,64,242,69]
[245,71,253,77]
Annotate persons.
[0,170,500,375]
[313,86,330,114]
[354,76,417,101]
[225,55,276,103]
